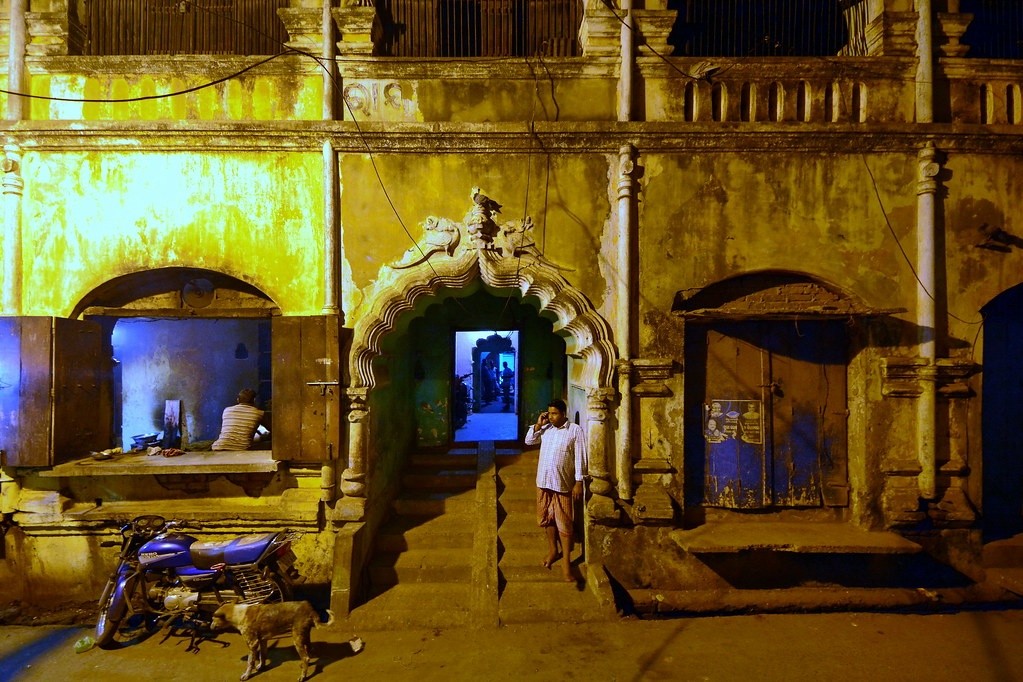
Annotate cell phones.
[544,414,548,419]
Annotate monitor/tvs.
[162,399,188,451]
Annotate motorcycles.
[457,372,474,429]
[94,513,307,647]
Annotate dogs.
[209,600,333,682]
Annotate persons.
[525,398,589,583]
[705,419,722,438]
[481,358,515,411]
[743,401,760,419]
[709,402,723,417]
[210,388,264,452]
